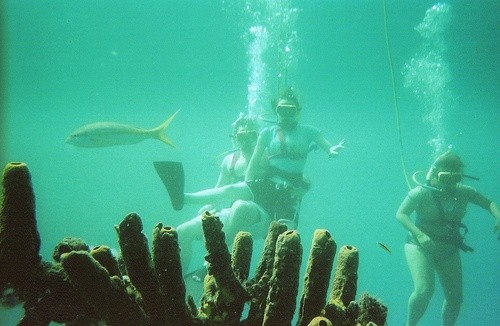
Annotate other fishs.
[64,107,181,152]
[376,240,394,255]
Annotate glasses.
[276,105,297,116]
[236,130,258,142]
[438,172,461,184]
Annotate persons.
[395,153,500,326]
[153,92,345,233]
[175,116,271,282]
